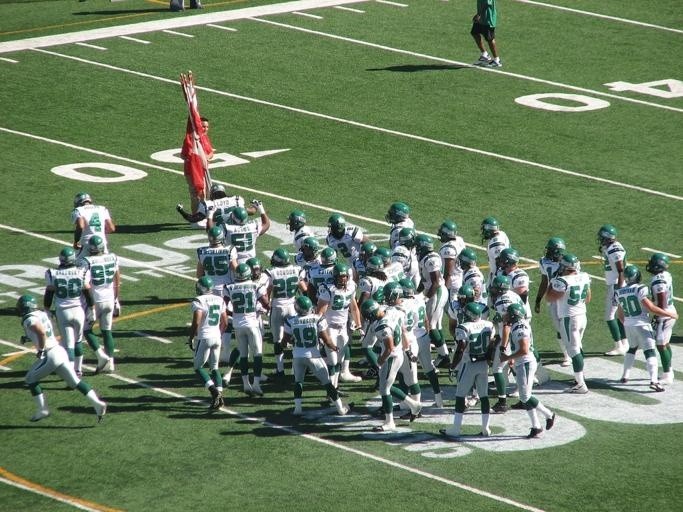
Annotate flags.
[179,73,213,196]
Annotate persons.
[179,116,217,231]
[468,0,503,68]
[167,0,207,11]
[15,294,106,425]
[72,190,121,325]
[76,234,121,375]
[43,246,97,380]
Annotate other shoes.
[562,358,571,367]
[659,373,675,385]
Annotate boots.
[330,382,339,389]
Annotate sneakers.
[95,401,108,424]
[222,370,285,396]
[604,345,629,357]
[369,346,554,440]
[340,370,363,383]
[93,359,115,374]
[75,370,84,378]
[291,407,303,416]
[336,406,349,415]
[621,375,627,382]
[484,59,502,68]
[474,53,491,65]
[569,380,577,385]
[206,394,226,413]
[29,409,50,422]
[649,381,665,392]
[565,383,588,394]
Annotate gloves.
[36,350,47,360]
[89,308,97,326]
[113,297,120,318]
[188,336,196,351]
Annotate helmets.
[231,207,249,225]
[16,294,38,314]
[207,225,225,244]
[270,247,291,267]
[209,184,226,198]
[289,202,521,322]
[73,192,94,207]
[544,237,567,260]
[623,264,642,286]
[58,246,76,266]
[87,235,104,254]
[558,253,581,275]
[646,252,672,276]
[597,223,617,242]
[233,257,261,282]
[195,275,214,296]
